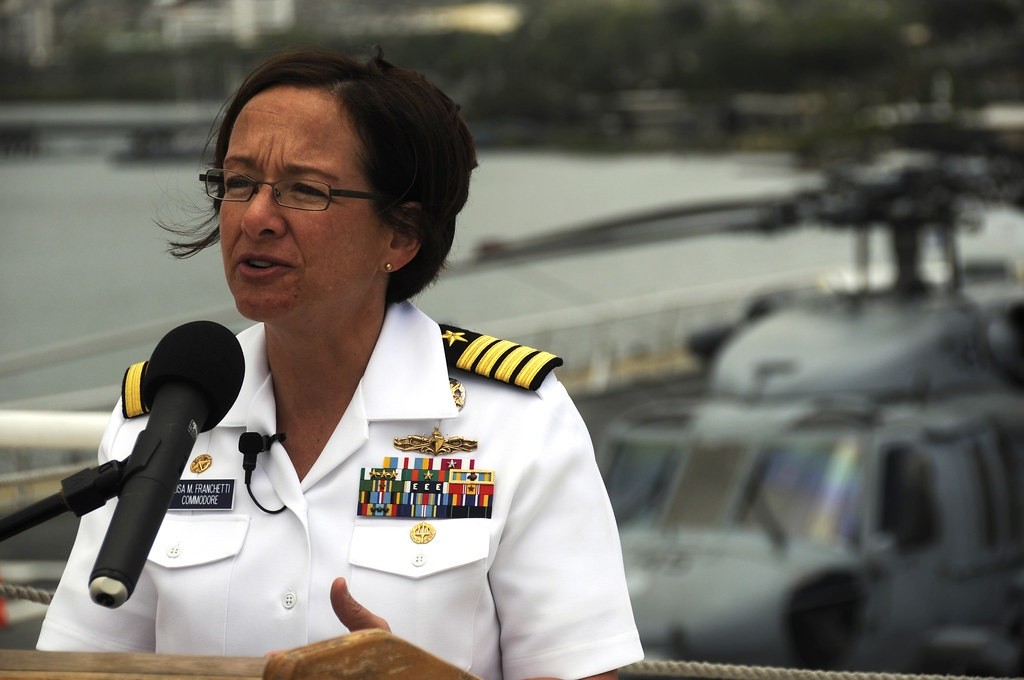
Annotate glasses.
[199,168,404,211]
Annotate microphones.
[88,321,246,609]
[238,431,265,484]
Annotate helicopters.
[433,144,1024,679]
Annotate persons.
[40,52,645,680]
